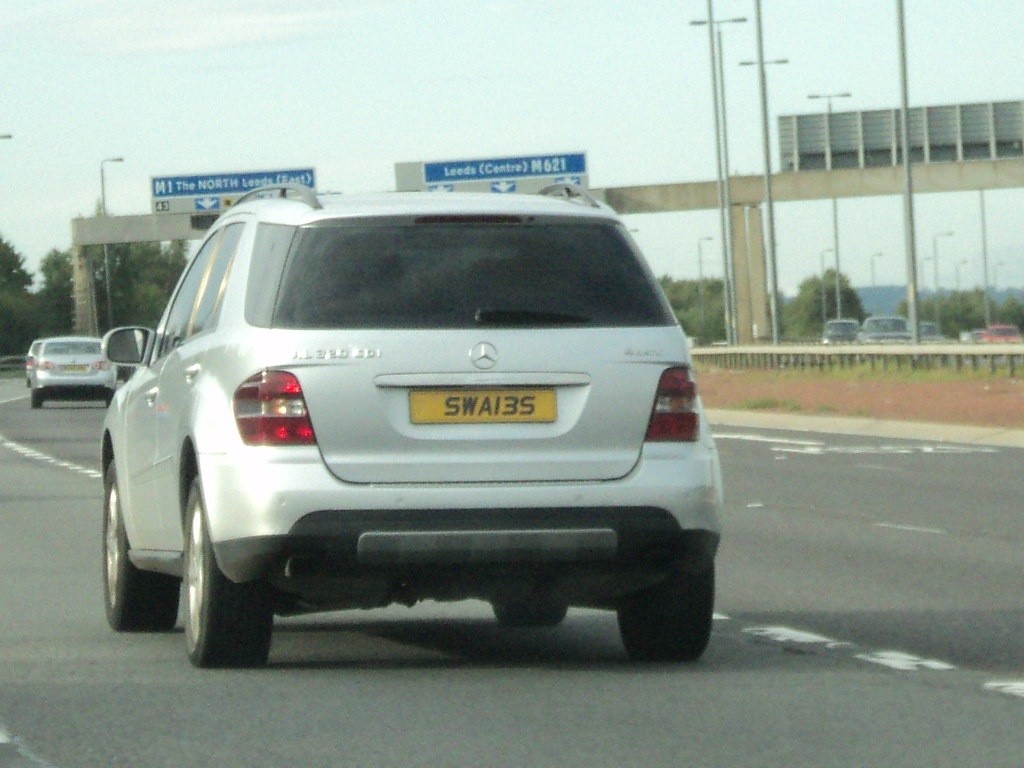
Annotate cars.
[916,321,1022,343]
[855,316,912,343]
[31,336,117,409]
[822,319,861,345]
[25,339,43,387]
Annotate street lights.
[808,93,853,318]
[698,236,714,324]
[933,231,955,293]
[689,16,746,348]
[738,57,790,344]
[100,157,125,329]
[870,254,884,290]
[819,248,835,317]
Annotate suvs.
[98,180,723,667]
